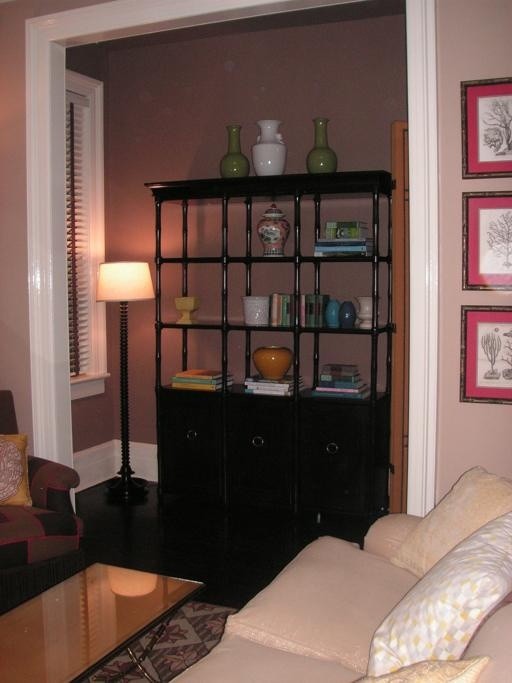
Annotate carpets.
[88,602,234,683]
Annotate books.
[171,368,235,392]
[311,364,372,401]
[314,220,373,258]
[244,374,305,397]
[270,292,329,329]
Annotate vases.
[220,117,338,177]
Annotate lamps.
[96,262,155,497]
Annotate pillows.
[355,466,512,683]
[0,434,32,507]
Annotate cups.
[243,296,270,325]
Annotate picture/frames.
[459,305,512,405]
[462,191,512,291]
[461,76,512,180]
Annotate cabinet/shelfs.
[143,170,392,571]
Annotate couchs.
[169,513,512,683]
[0,390,84,577]
